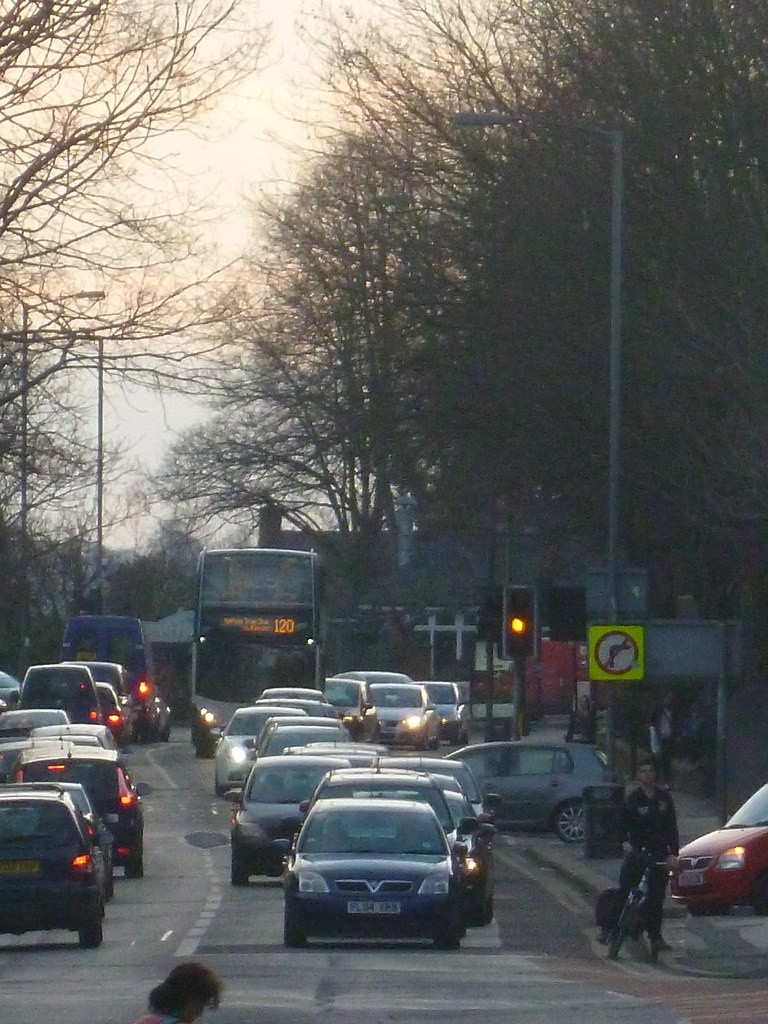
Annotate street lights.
[453,109,625,776]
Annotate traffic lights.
[505,584,534,658]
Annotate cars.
[669,779,767,917]
[442,740,627,844]
[270,798,470,950]
[0,666,466,885]
[0,784,106,949]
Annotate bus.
[189,547,324,760]
[59,614,155,746]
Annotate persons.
[596,758,679,952]
[136,961,220,1024]
[562,695,596,745]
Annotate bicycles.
[602,845,684,960]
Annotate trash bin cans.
[583,783,627,860]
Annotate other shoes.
[649,937,670,955]
[599,924,618,943]
